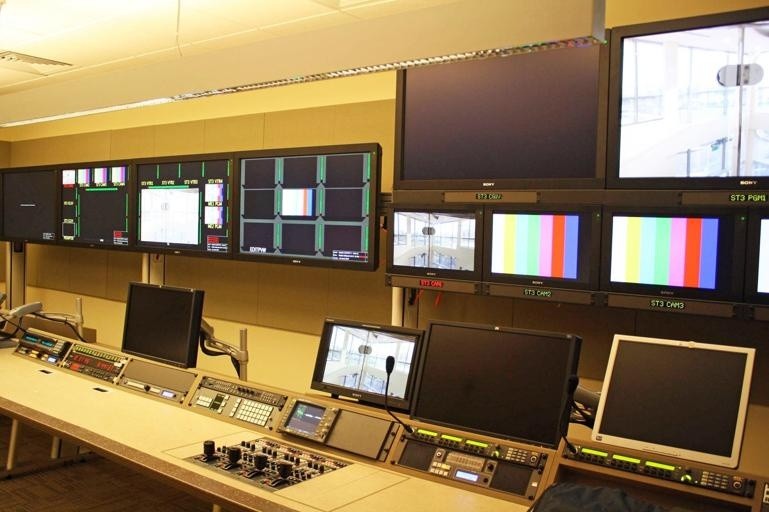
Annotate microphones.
[385,356,413,434]
[561,375,579,458]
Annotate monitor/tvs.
[393,39,598,192]
[232,142,382,271]
[598,7,769,190]
[132,152,232,260]
[483,204,600,291]
[57,159,133,252]
[0,159,58,251]
[600,208,745,301]
[746,206,769,304]
[410,319,582,448]
[310,317,425,412]
[385,203,482,280]
[590,333,756,469]
[121,281,205,369]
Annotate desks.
[1,325,540,512]
